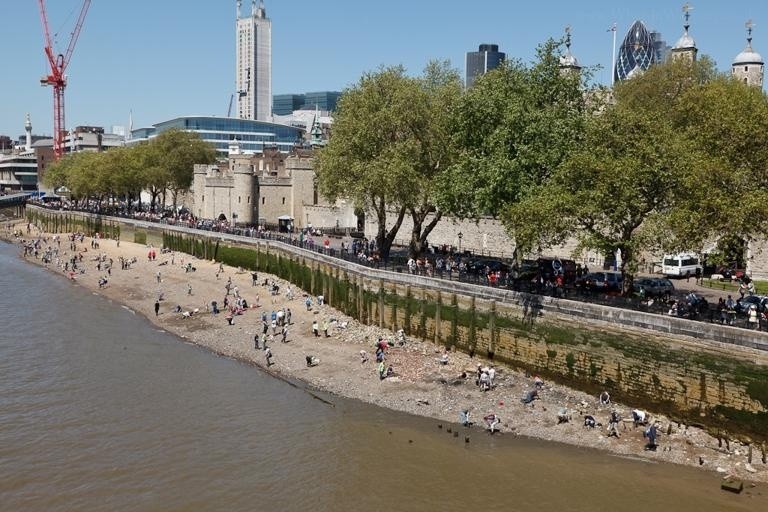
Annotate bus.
[662,254,703,278]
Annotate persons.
[8,201,757,448]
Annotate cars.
[467,257,675,296]
[733,295,768,315]
